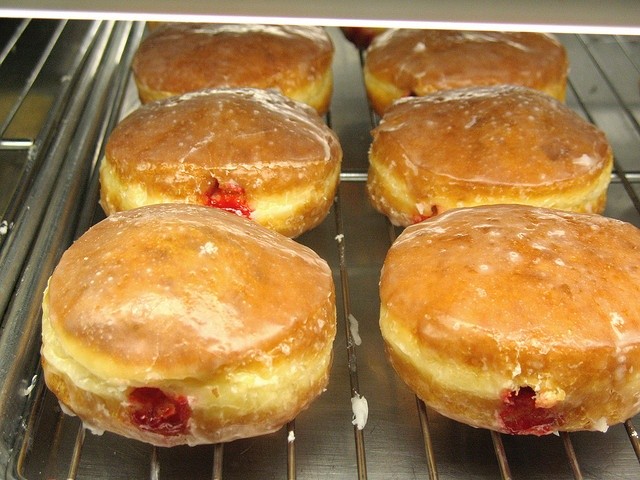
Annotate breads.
[341,27,384,50]
[377,204,639,436]
[39,204,336,450]
[97,88,343,237]
[362,28,570,119]
[366,84,614,227]
[130,22,334,119]
